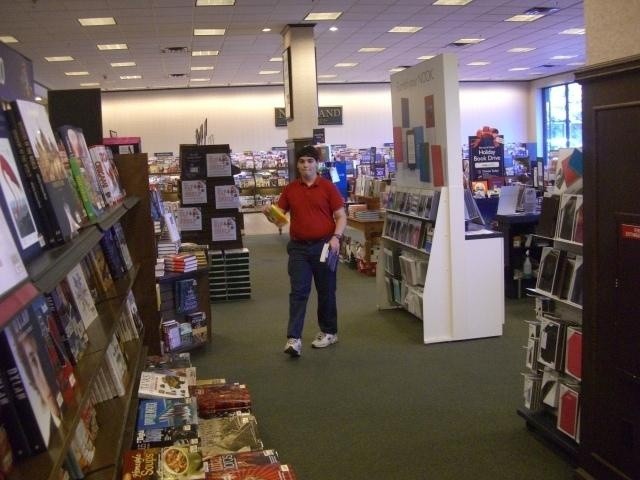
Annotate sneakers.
[283,338,302,356]
[311,331,339,348]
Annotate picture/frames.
[275,106,343,127]
[283,48,291,126]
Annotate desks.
[465,228,508,344]
[497,213,538,298]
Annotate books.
[232,151,291,208]
[148,153,240,350]
[464,144,583,437]
[1,97,145,479]
[122,352,297,480]
[382,182,440,320]
[328,143,397,221]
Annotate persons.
[260,145,347,357]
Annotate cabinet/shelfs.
[517,189,585,457]
[229,153,296,215]
[1,111,151,476]
[149,156,179,204]
[378,185,463,345]
[151,215,218,352]
[338,177,385,277]
